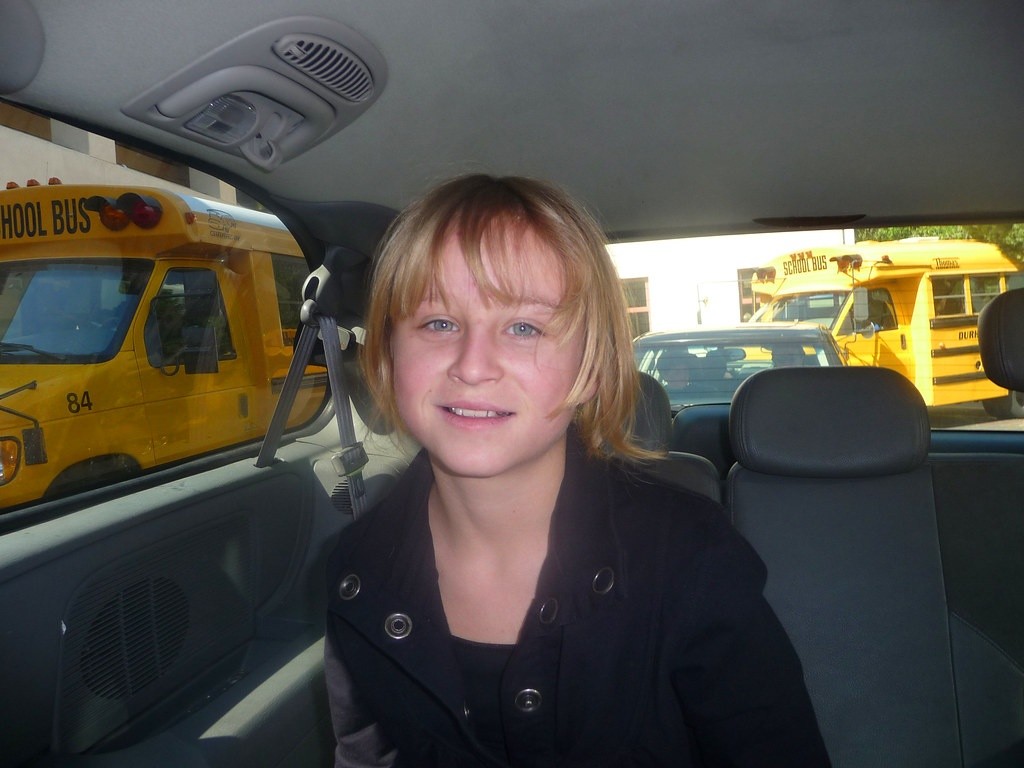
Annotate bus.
[0,177,327,516]
[729,234,1024,419]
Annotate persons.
[654,342,809,400]
[320,177,835,768]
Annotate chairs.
[617,286,1024,767]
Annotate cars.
[631,325,850,413]
